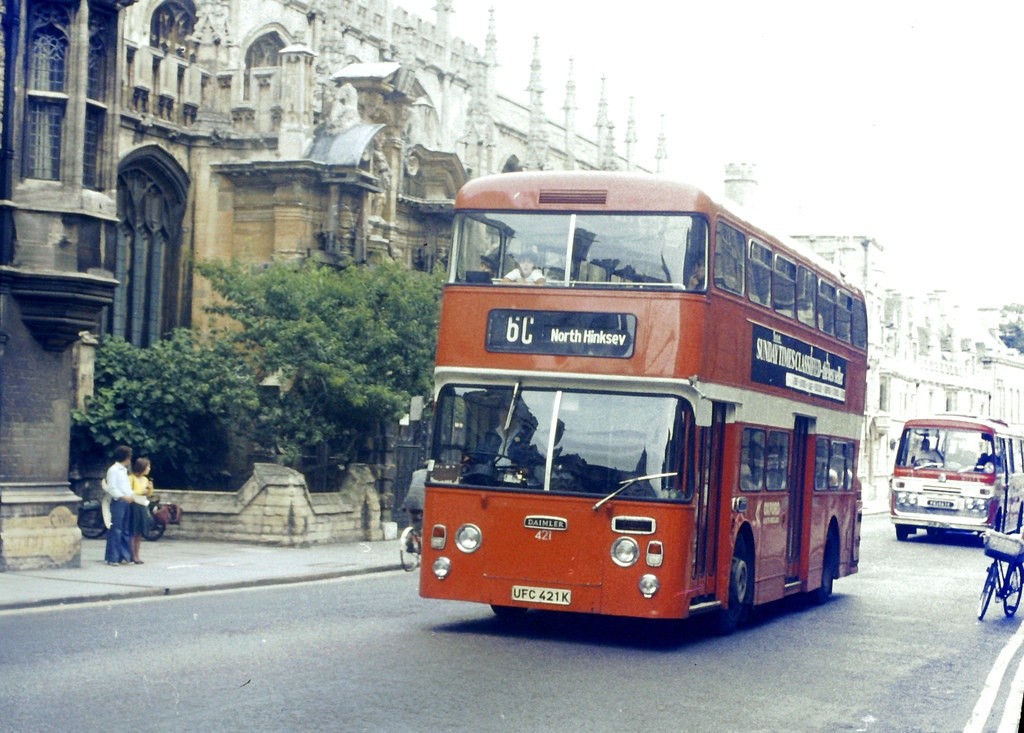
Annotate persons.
[686,253,742,292]
[401,467,428,549]
[105,445,136,565]
[462,402,534,468]
[916,438,942,466]
[128,458,154,564]
[501,248,546,285]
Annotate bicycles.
[398,512,424,574]
[975,531,1024,620]
[79,493,167,542]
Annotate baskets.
[984,535,1023,563]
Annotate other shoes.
[405,542,415,551]
[108,558,134,566]
[130,557,143,565]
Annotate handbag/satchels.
[154,502,183,525]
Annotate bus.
[888,415,1024,539]
[399,169,869,637]
